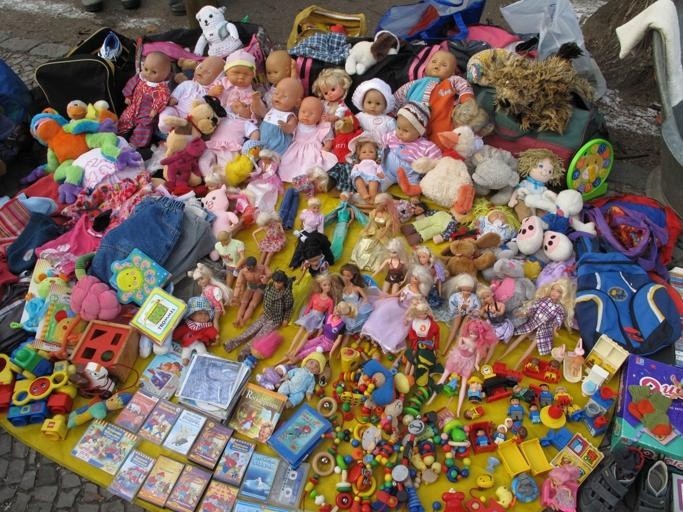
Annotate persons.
[246,149,283,216]
[252,212,285,268]
[158,56,224,135]
[311,69,360,162]
[359,264,433,355]
[403,301,440,378]
[118,50,171,146]
[288,246,329,327]
[381,100,442,202]
[186,262,233,334]
[352,77,396,143]
[287,197,334,271]
[441,276,480,356]
[288,301,358,362]
[278,167,328,230]
[498,281,568,371]
[214,231,244,287]
[230,257,271,329]
[285,273,333,355]
[344,131,384,204]
[394,50,474,148]
[208,51,266,119]
[260,50,297,110]
[479,287,514,365]
[222,271,293,361]
[173,297,220,366]
[369,237,408,296]
[242,77,303,159]
[427,319,496,418]
[324,191,368,264]
[276,97,339,184]
[351,192,410,274]
[409,247,445,302]
[278,353,327,408]
[336,263,373,360]
[239,332,284,369]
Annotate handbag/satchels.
[374,0,485,43]
[286,4,367,50]
[35,27,136,118]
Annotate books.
[70,352,332,512]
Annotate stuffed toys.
[201,184,239,261]
[159,95,228,191]
[397,125,597,292]
[193,5,242,60]
[20,98,142,203]
[343,31,400,76]
[227,185,254,235]
